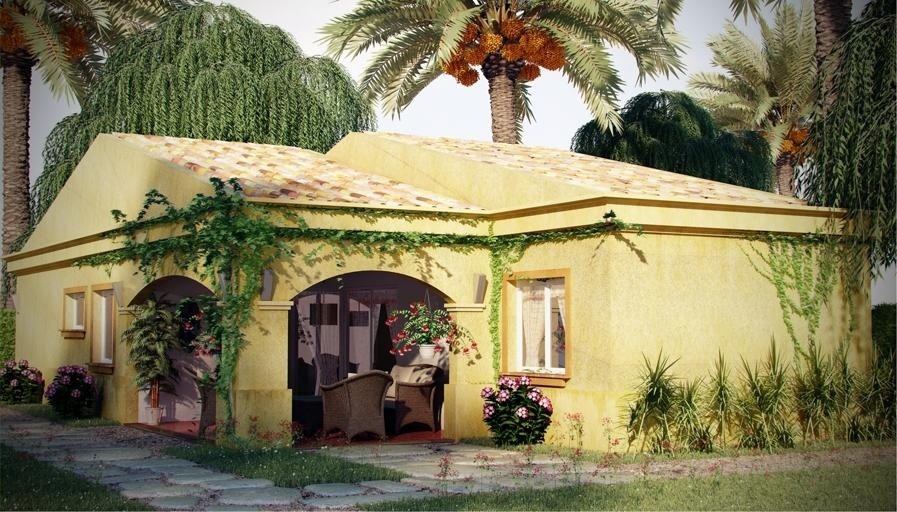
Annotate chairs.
[319,369,394,444]
[185,365,216,437]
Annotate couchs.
[384,364,444,434]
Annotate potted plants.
[119,291,183,426]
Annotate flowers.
[385,301,478,358]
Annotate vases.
[416,343,437,360]
